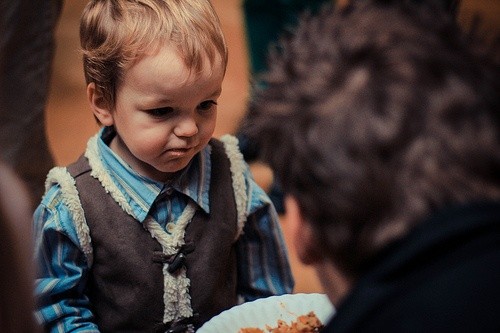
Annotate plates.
[195,293,336,333]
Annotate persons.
[238,0,322,213]
[1,1,62,213]
[247,1,500,333]
[28,0,295,333]
